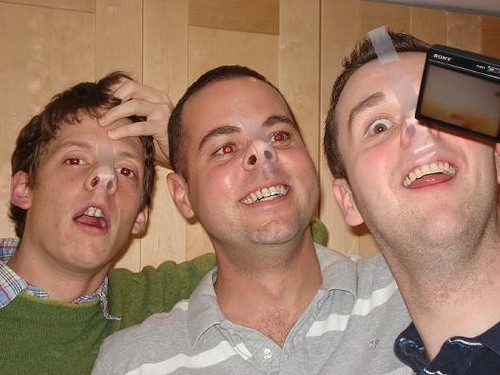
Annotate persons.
[322,30,500,375]
[0,71,329,375]
[91,62,411,375]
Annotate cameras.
[414,43,500,144]
